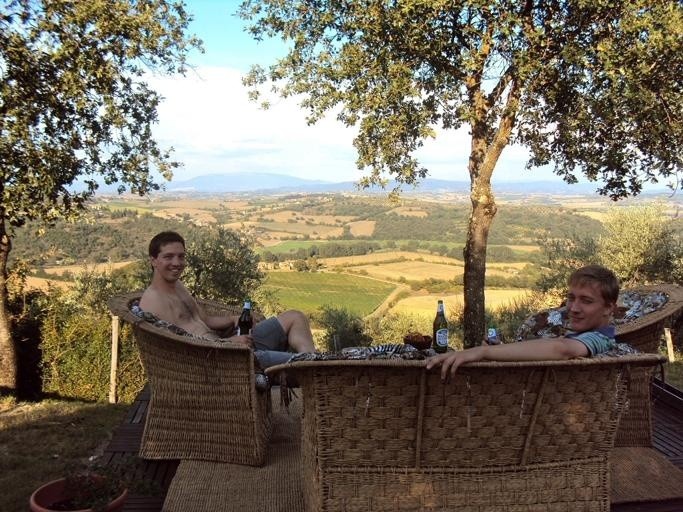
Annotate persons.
[139,231,317,367]
[425,264,619,381]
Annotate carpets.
[161,385,682,511]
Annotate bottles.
[238,296,253,335]
[488,325,498,346]
[434,300,448,354]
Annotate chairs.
[547,284,683,447]
[106,290,278,468]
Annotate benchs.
[260,353,669,511]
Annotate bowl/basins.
[404,336,432,351]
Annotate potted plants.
[26,453,164,512]
